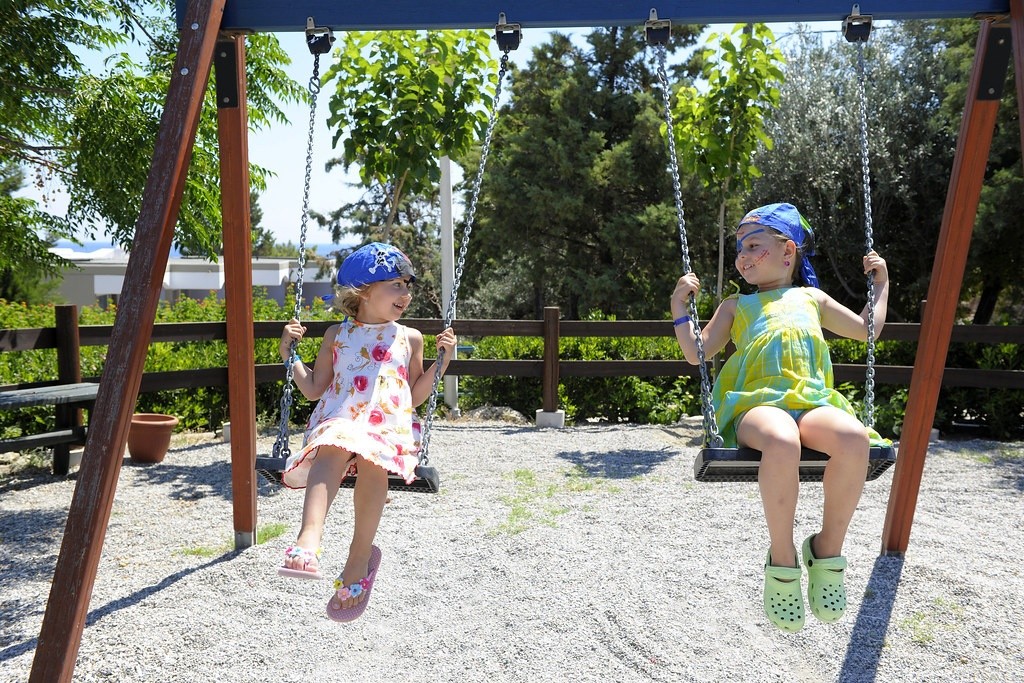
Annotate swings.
[253,12,523,494]
[643,4,897,484]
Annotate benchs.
[0,380,101,481]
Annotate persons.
[278,243,458,624]
[670,202,888,631]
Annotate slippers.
[278,544,324,582]
[763,542,805,633]
[802,533,847,623]
[325,543,381,624]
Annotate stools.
[255,435,439,496]
[693,422,899,489]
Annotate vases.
[126,413,180,462]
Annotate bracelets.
[282,355,302,370]
[672,314,692,327]
